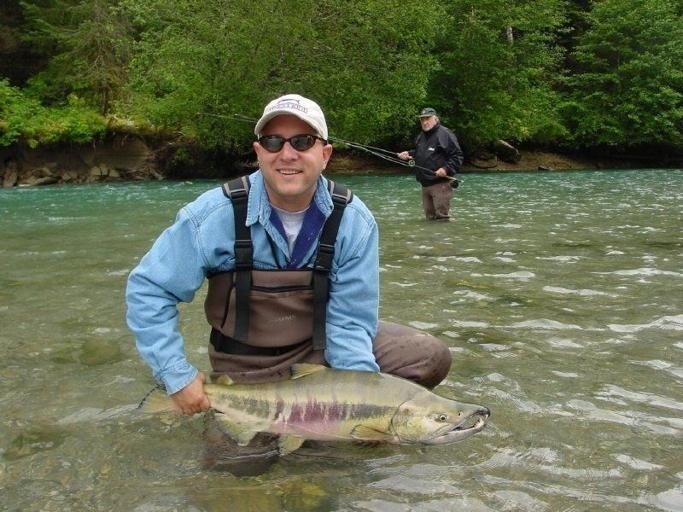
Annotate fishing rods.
[194,112,465,183]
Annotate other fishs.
[131,362,494,458]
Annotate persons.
[398,107,464,221]
[126,93,451,477]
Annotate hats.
[418,108,435,117]
[254,94,328,141]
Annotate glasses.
[258,134,327,152]
